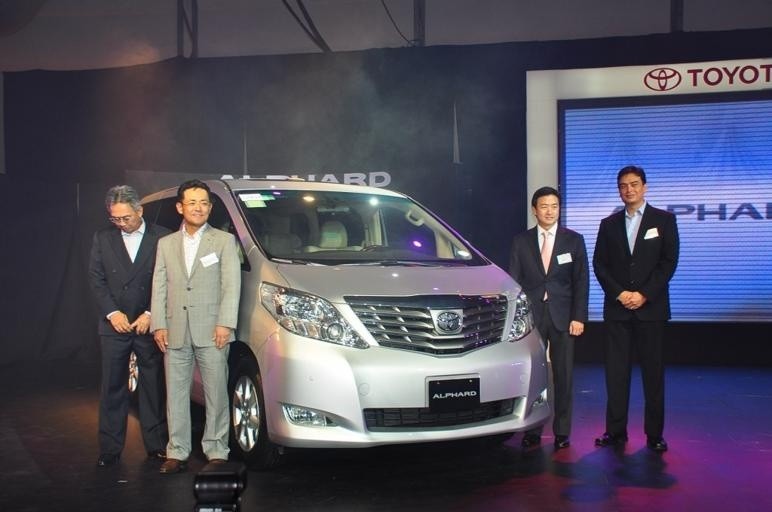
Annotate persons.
[147,178,243,478]
[592,164,681,452]
[508,187,590,449]
[87,182,168,482]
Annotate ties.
[541,232,550,301]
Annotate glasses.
[181,201,211,209]
[108,213,134,224]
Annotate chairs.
[221,197,366,265]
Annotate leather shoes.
[97,452,120,466]
[202,459,228,471]
[522,434,542,446]
[553,436,570,447]
[647,438,667,450]
[146,451,166,465]
[594,433,628,445]
[159,458,188,473]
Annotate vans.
[129,179,555,478]
